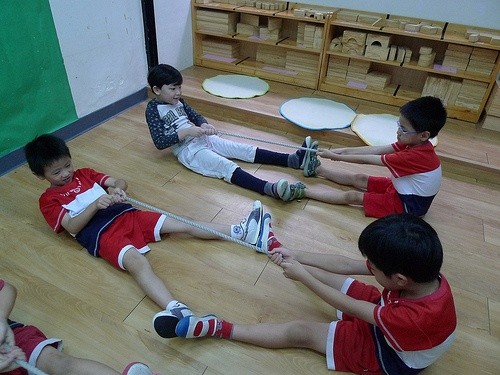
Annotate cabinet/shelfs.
[190,0,339,92]
[317,6,500,124]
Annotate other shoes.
[174,313,218,339]
[122,361,152,375]
[256,213,273,254]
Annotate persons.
[23,134,263,339]
[144,64,312,200]
[0,278,154,375]
[175,212,457,375]
[280,96,448,220]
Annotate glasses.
[397,121,422,135]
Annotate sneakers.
[152,301,194,339]
[276,178,288,199]
[240,199,264,245]
[283,181,307,203]
[296,136,312,169]
[303,140,321,177]
